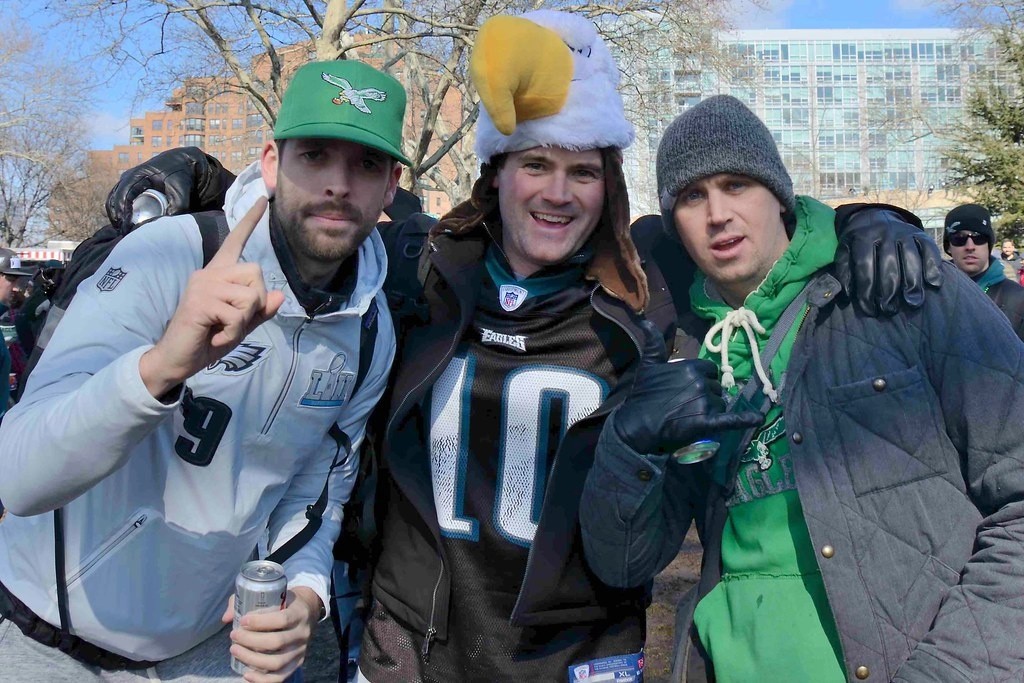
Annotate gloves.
[836,206,946,318]
[613,319,766,455]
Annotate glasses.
[947,231,989,247]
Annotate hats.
[274,60,413,168]
[656,94,796,244]
[943,203,995,256]
[0,249,33,276]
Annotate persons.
[0,58,1024,683]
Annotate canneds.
[230,560,288,675]
[126,188,167,225]
